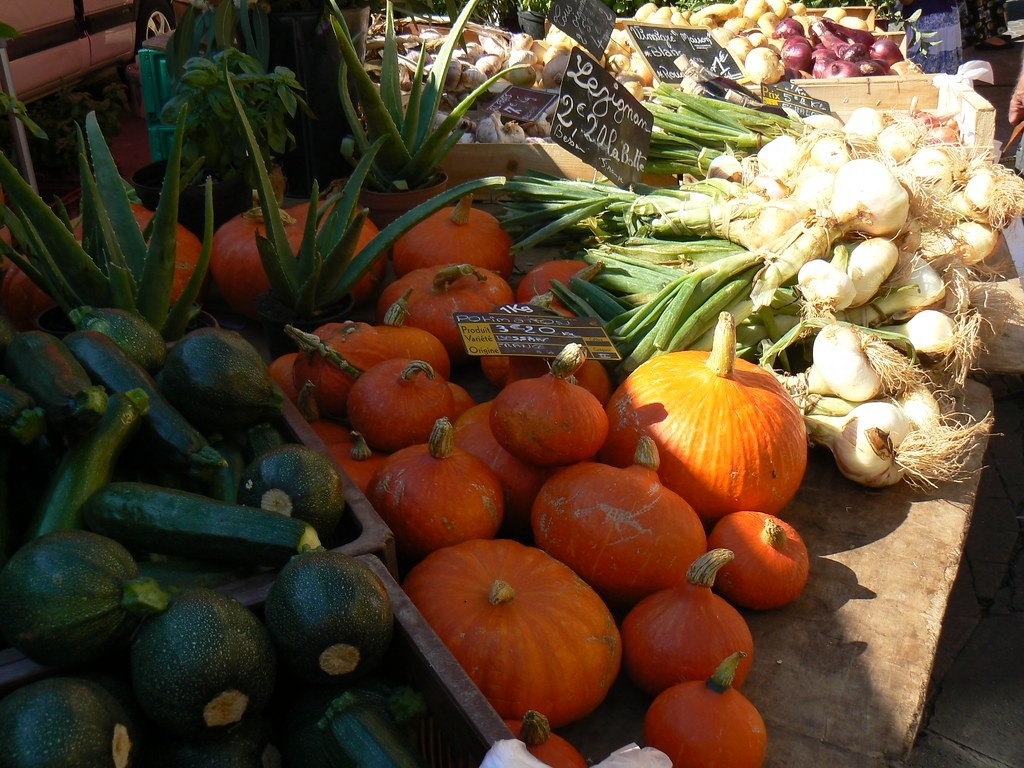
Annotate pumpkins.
[1,197,809,768]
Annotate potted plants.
[0,100,223,354]
[328,0,535,257]
[223,53,507,357]
[128,48,318,242]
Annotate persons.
[1009,46,1024,179]
[956,0,1015,51]
[900,0,963,74]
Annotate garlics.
[373,27,653,145]
[709,105,1024,490]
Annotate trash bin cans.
[129,156,221,241]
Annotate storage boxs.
[0,331,527,768]
[438,7,940,194]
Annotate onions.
[708,17,927,83]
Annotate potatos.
[634,0,868,32]
